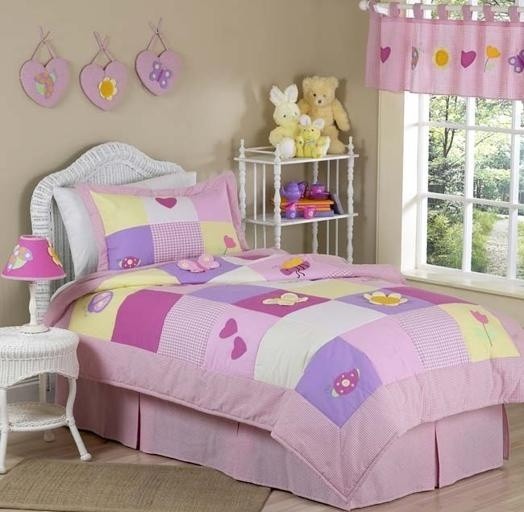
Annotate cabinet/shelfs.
[234,135,359,264]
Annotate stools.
[0,327,91,474]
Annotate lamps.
[0,234,65,333]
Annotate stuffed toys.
[267,84,331,161]
[294,113,325,159]
[296,74,351,154]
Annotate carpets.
[0,455,271,512]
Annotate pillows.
[50,172,197,280]
[81,169,245,272]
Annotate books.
[270,194,335,219]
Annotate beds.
[29,141,524,512]
